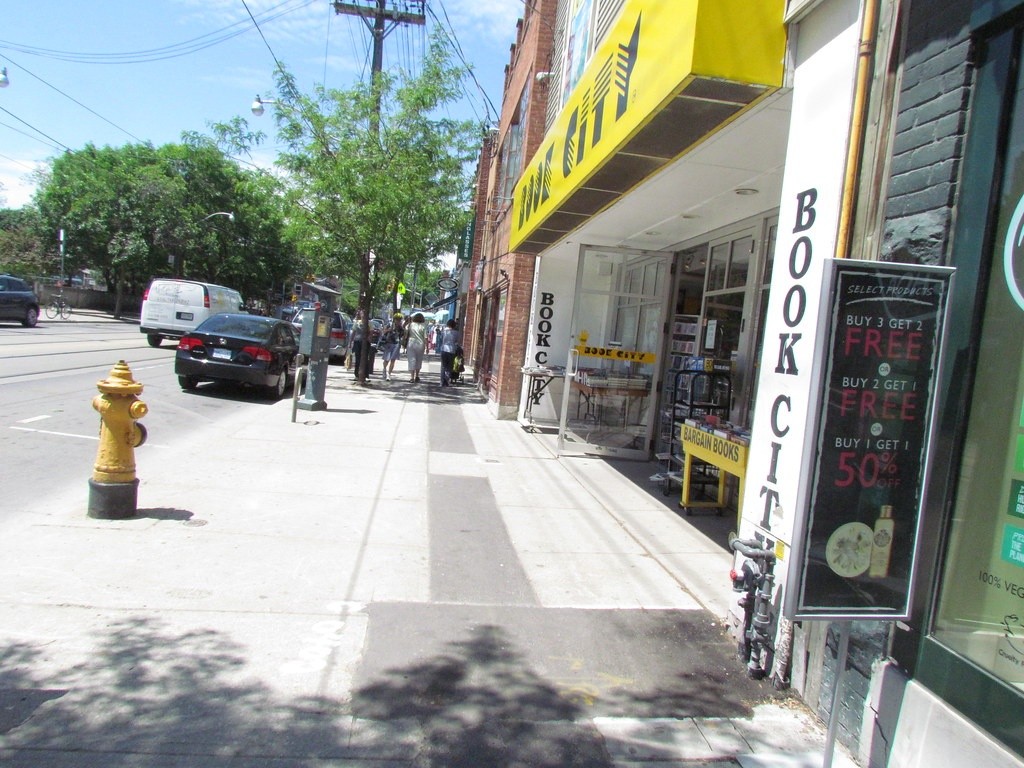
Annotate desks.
[681,424,749,536]
[520,372,648,434]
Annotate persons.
[348,312,460,388]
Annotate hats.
[394,313,403,320]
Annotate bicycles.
[46,294,73,320]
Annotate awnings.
[421,293,457,312]
[306,283,342,297]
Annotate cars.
[370,321,383,345]
[175,313,309,401]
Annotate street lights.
[201,211,236,223]
[251,94,304,116]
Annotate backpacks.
[385,322,401,344]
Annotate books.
[521,364,652,391]
[661,322,753,447]
[648,452,718,485]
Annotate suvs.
[0,274,40,328]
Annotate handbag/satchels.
[402,324,411,347]
[345,348,353,369]
[453,356,465,372]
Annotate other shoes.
[415,377,420,382]
[386,374,390,381]
[441,377,450,386]
[364,377,371,382]
[354,377,358,380]
[383,370,386,377]
[409,379,414,383]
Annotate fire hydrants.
[86,360,147,520]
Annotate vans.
[292,308,355,364]
[139,277,248,349]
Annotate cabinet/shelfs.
[662,370,732,501]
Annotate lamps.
[699,255,707,265]
[685,255,694,269]
[477,287,484,295]
[481,195,514,232]
[500,269,508,277]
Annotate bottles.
[869,505,894,577]
